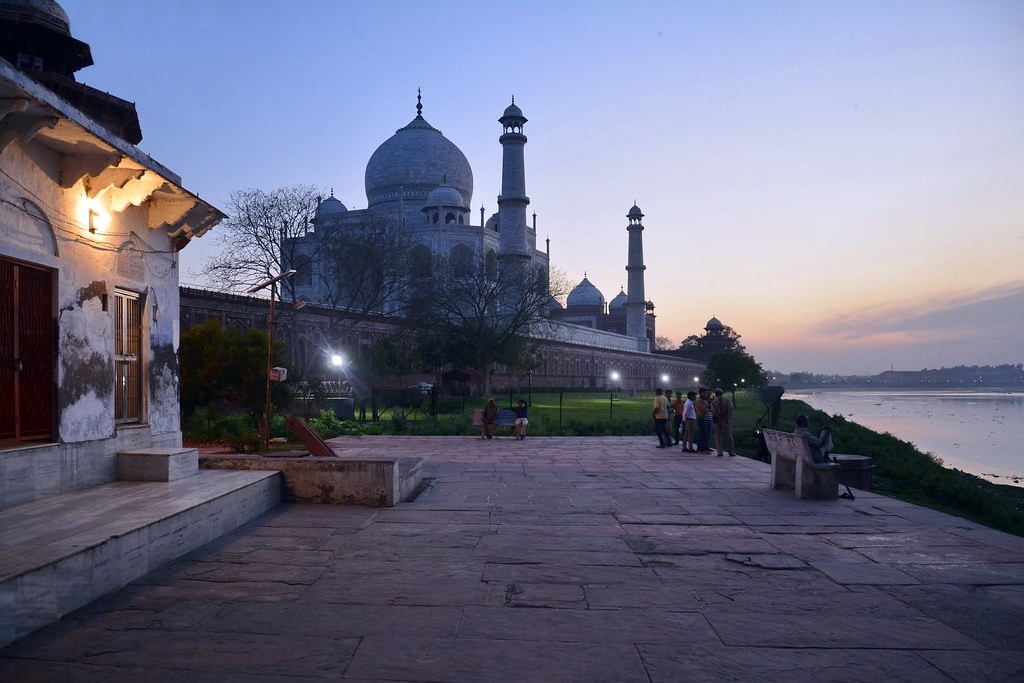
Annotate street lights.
[527,370,532,406]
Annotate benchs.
[761,427,841,501]
[473,409,527,440]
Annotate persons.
[794,414,834,464]
[652,387,737,457]
[480,398,499,440]
[500,400,529,440]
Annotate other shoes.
[488,434,492,440]
[656,445,665,448]
[706,448,714,451]
[717,454,723,456]
[730,453,736,456]
[688,449,696,453]
[672,443,679,445]
[696,449,705,452]
[519,434,524,441]
[522,433,525,438]
[682,448,689,452]
[664,444,673,447]
[486,432,490,437]
